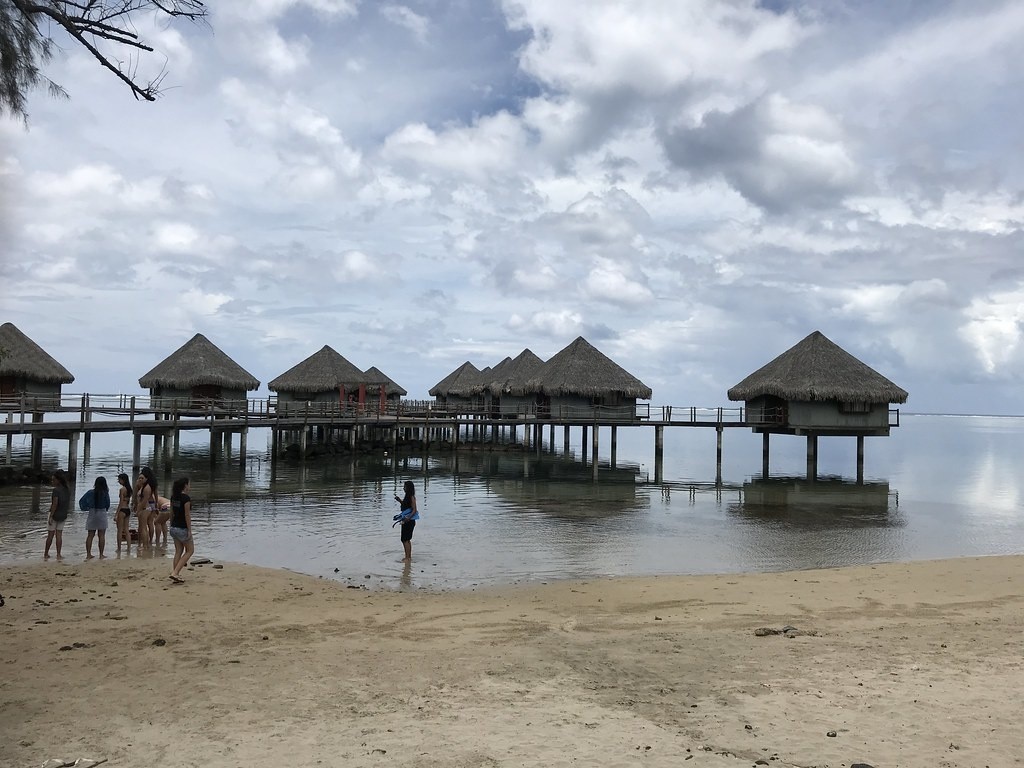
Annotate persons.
[114,466,170,549]
[44,472,71,560]
[168,478,194,584]
[79,476,110,560]
[394,481,416,563]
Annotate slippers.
[173,580,182,585]
[169,575,185,582]
